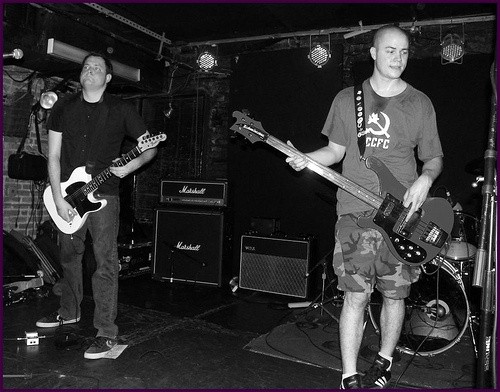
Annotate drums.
[369,256,470,356]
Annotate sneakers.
[35,311,80,328]
[84,337,117,359]
[339,372,360,389]
[361,352,392,388]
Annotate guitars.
[439,213,481,260]
[230,111,454,265]
[43,132,167,232]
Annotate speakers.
[239,235,310,298]
[153,209,237,288]
[0,230,55,300]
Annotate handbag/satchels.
[8,152,49,181]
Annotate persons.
[36,52,157,359]
[286,24,443,389]
